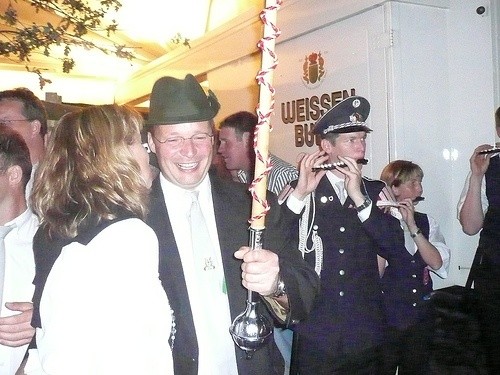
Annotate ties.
[335,181,347,205]
[401,220,415,256]
[189,190,236,375]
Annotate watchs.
[355,196,371,213]
[270,280,287,298]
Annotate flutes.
[398,196,426,210]
[310,158,368,172]
[477,148,500,155]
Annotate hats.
[311,96,374,134]
[144,73,220,124]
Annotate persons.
[277,95,406,375]
[36,103,175,375]
[377,160,451,332]
[146,74,321,375]
[457,107,500,302]
[218,111,300,196]
[0,87,49,203]
[0,125,43,375]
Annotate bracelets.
[411,228,422,239]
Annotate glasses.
[152,133,214,149]
